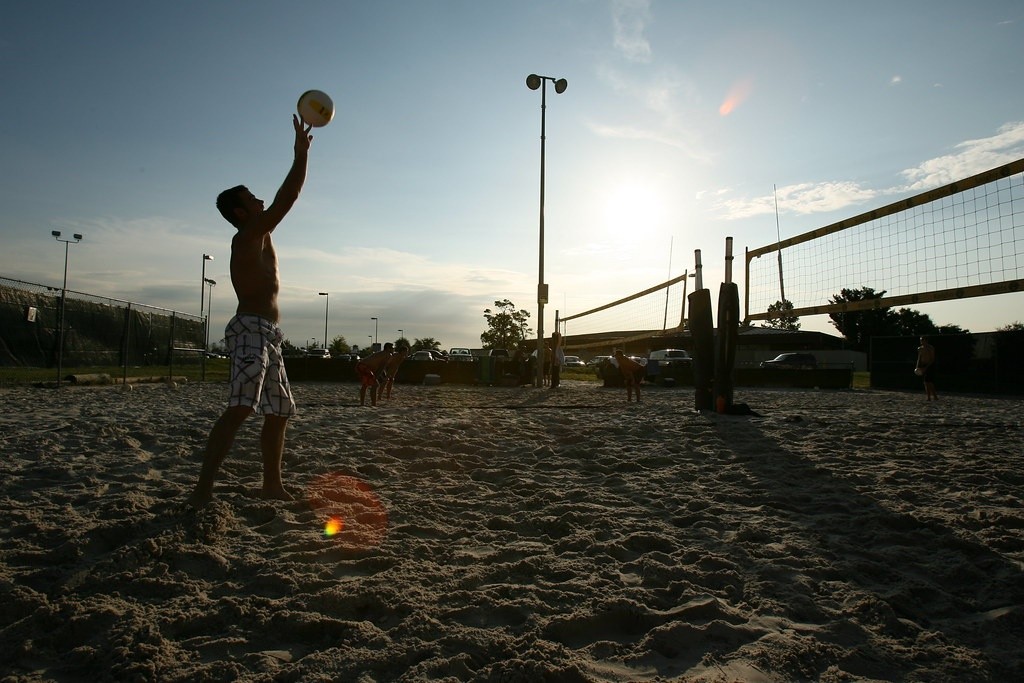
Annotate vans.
[649,349,693,368]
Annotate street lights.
[200,253,214,322]
[52,230,83,388]
[205,277,217,351]
[319,292,328,349]
[525,73,568,388]
[398,330,403,340]
[371,317,378,344]
[368,335,372,346]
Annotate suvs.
[308,348,331,359]
[488,348,509,358]
[760,352,818,371]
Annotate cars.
[587,355,648,369]
[407,348,448,362]
[336,353,361,362]
[563,355,586,368]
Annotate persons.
[543,340,565,389]
[513,346,526,375]
[613,349,644,403]
[915,336,939,401]
[181,113,314,512]
[355,343,409,406]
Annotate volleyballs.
[178,377,187,384]
[168,381,177,388]
[296,90,336,127]
[914,368,921,375]
[121,384,132,391]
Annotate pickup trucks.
[445,347,474,363]
